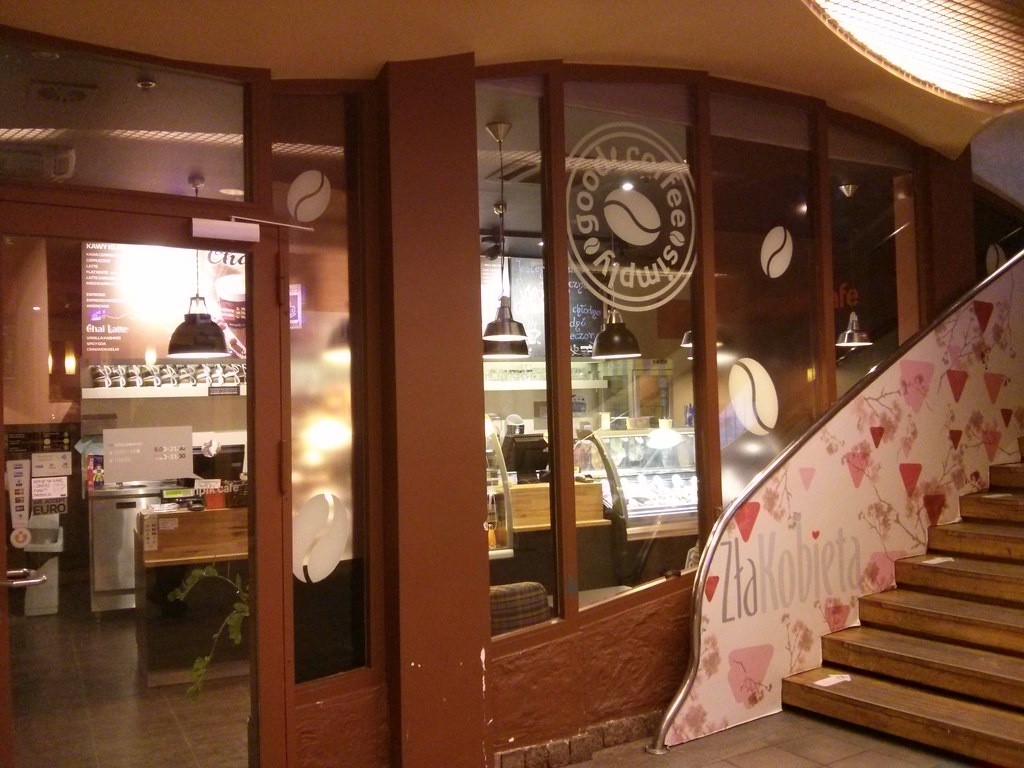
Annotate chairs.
[490,582,552,637]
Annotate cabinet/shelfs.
[133,506,250,687]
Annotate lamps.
[591,232,642,360]
[835,185,873,346]
[168,173,230,359]
[681,331,724,360]
[482,122,530,359]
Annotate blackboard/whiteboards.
[508,256,606,358]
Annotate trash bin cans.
[22,526,65,616]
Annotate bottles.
[488,524,496,549]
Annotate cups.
[659,419,673,428]
